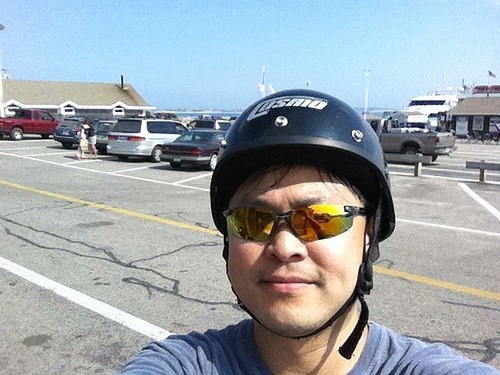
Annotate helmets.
[209,88,397,243]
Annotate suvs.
[106,115,201,163]
[188,114,237,130]
[53,115,106,148]
[96,120,116,154]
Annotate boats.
[400,78,469,138]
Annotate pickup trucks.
[0,109,61,141]
[366,118,458,165]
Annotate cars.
[159,129,229,170]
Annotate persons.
[75,117,98,160]
[119,89,500,375]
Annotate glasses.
[221,202,367,244]
